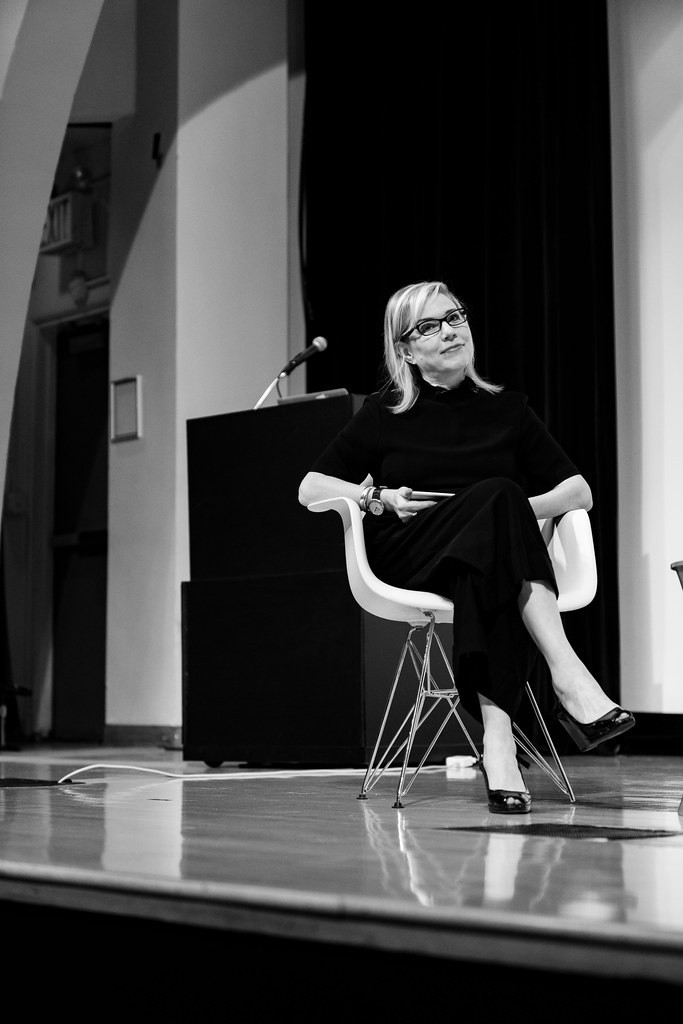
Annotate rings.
[413,512,417,516]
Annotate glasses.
[401,306,469,341]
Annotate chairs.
[307,496,598,808]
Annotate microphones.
[279,336,326,379]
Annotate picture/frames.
[109,373,142,441]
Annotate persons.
[298,282,636,813]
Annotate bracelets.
[360,485,374,512]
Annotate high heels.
[557,702,640,751]
[477,755,539,814]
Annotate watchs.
[368,486,388,516]
